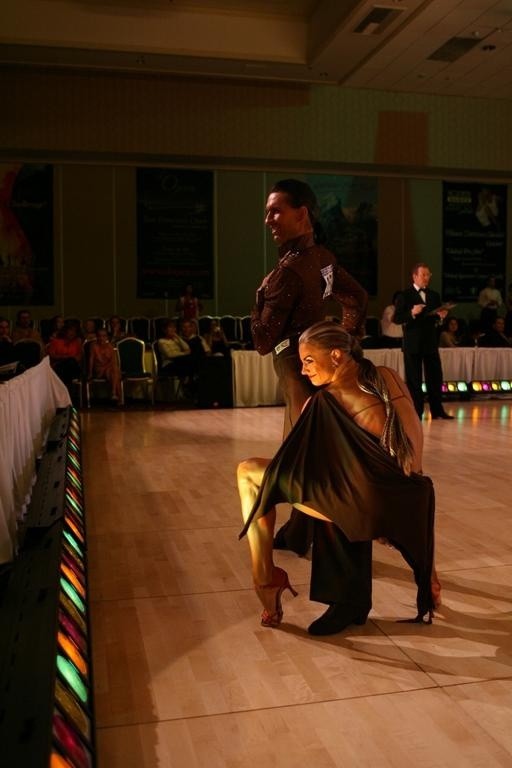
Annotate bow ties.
[418,288,428,293]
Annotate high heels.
[430,581,441,609]
[252,565,298,626]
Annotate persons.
[82,319,122,397]
[234,320,443,629]
[438,317,465,347]
[381,291,406,348]
[175,284,205,321]
[477,278,502,332]
[358,295,377,347]
[107,316,132,345]
[489,316,508,347]
[46,315,84,395]
[391,262,455,422]
[157,320,220,397]
[203,319,232,372]
[3,310,46,381]
[241,176,373,637]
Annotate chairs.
[0,316,511,409]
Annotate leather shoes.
[432,415,454,420]
[309,605,372,636]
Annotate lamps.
[0,404,95,768]
